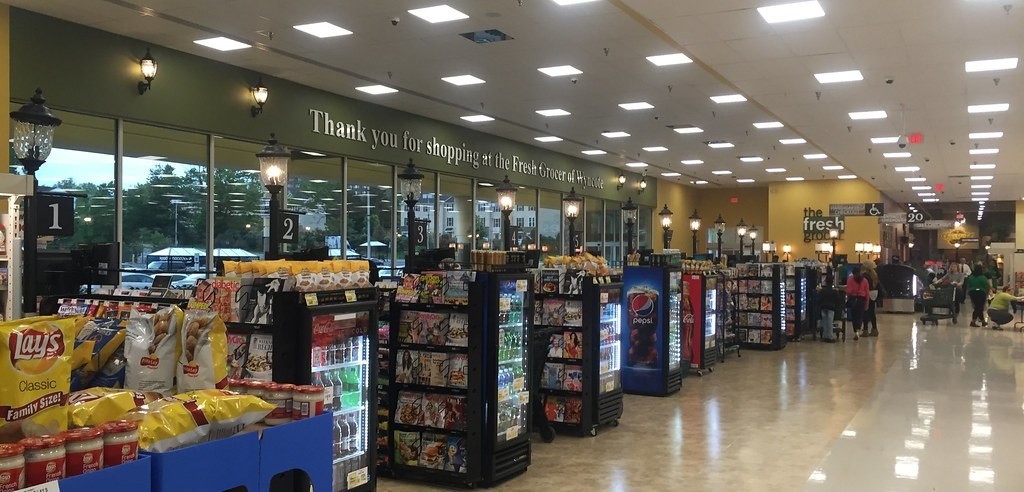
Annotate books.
[529,268,587,423]
[392,268,475,472]
[191,277,294,383]
[718,264,796,346]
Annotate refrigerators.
[621,253,683,396]
[683,271,718,376]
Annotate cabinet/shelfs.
[376,282,481,489]
[739,265,781,352]
[786,267,800,342]
[56,291,302,386]
[535,275,593,436]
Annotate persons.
[403,275,414,290]
[400,351,413,383]
[565,332,579,359]
[819,260,882,343]
[932,257,1024,331]
[547,338,562,357]
[786,292,794,305]
[245,281,275,324]
[761,296,771,310]
[197,282,210,301]
[761,331,772,344]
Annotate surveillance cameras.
[391,17,400,25]
[898,135,907,148]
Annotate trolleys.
[920,283,961,325]
[813,289,846,343]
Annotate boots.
[869,327,878,336]
[860,329,869,336]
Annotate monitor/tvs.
[300,246,328,261]
[727,255,756,269]
[638,249,654,265]
[405,247,456,273]
[695,254,713,262]
[36,241,120,297]
[526,250,540,269]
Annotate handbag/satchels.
[845,296,859,309]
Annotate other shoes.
[992,325,1004,330]
[825,337,837,342]
[982,320,988,326]
[853,331,860,340]
[970,321,980,327]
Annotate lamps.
[494,174,519,251]
[255,131,293,261]
[713,214,727,263]
[250,76,268,118]
[688,209,702,260]
[396,157,426,273]
[617,171,647,194]
[659,203,674,249]
[735,219,748,263]
[562,187,582,257]
[8,87,64,316]
[622,197,637,255]
[748,224,882,263]
[137,47,157,95]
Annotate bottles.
[311,368,359,411]
[332,413,358,460]
[499,293,522,324]
[600,323,615,346]
[498,330,521,361]
[683,282,694,361]
[600,303,613,319]
[705,315,711,334]
[662,249,680,262]
[599,348,614,374]
[311,336,359,367]
[705,289,712,310]
[498,363,524,401]
[497,400,520,433]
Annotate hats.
[862,258,877,268]
